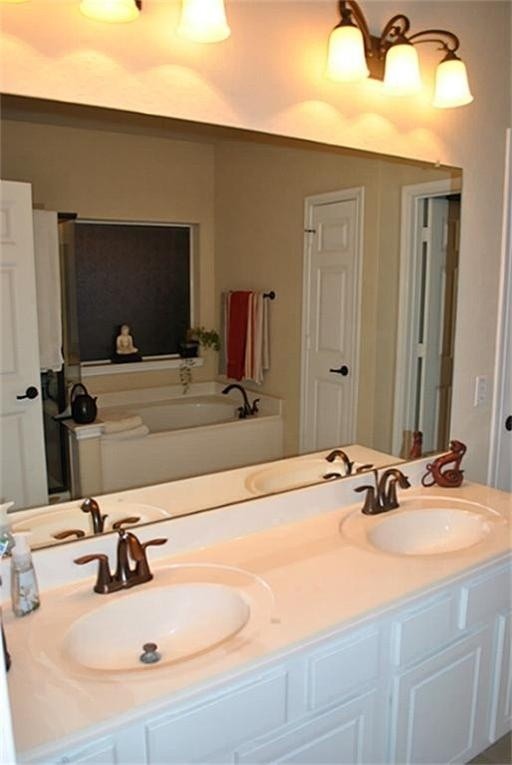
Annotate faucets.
[221,384,249,414]
[79,498,102,535]
[325,450,351,475]
[376,468,412,508]
[115,527,145,583]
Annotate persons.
[115,325,140,354]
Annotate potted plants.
[178,326,220,394]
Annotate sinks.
[339,496,509,561]
[12,499,170,547]
[52,562,274,685]
[244,460,368,498]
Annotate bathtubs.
[97,393,285,495]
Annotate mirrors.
[0,92,463,560]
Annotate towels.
[32,207,64,373]
[225,292,270,383]
[98,414,149,440]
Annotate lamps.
[327,0,474,108]
[80,0,231,42]
